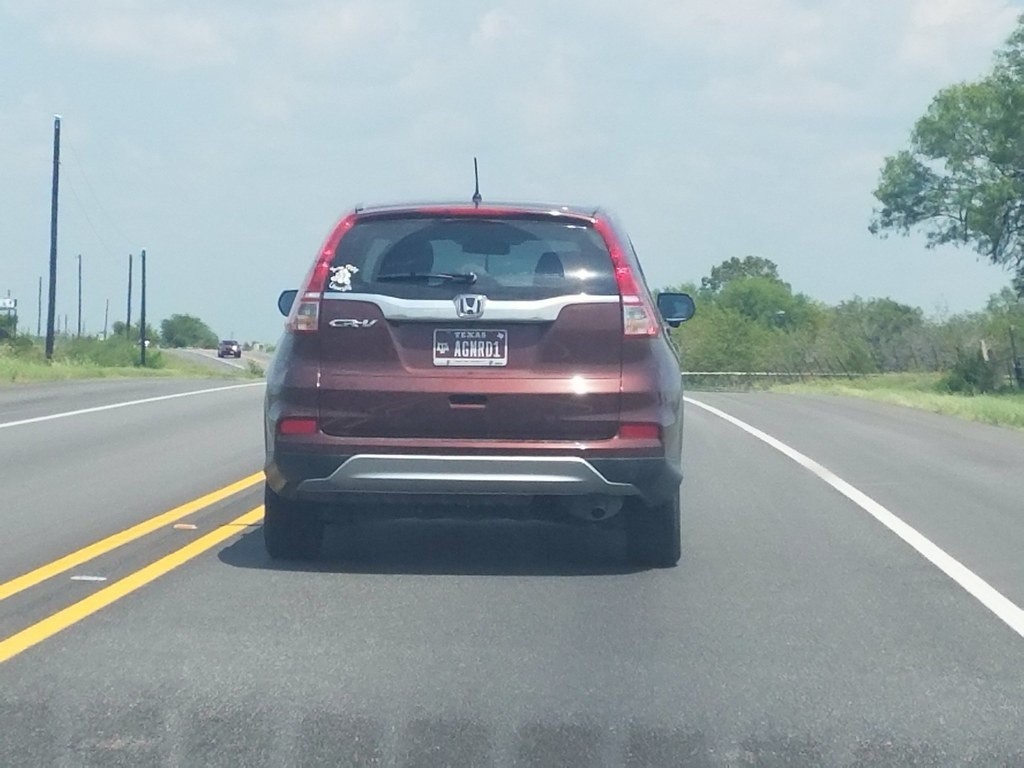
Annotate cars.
[217,339,243,359]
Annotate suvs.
[262,198,697,569]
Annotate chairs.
[371,243,430,285]
[533,252,593,287]
